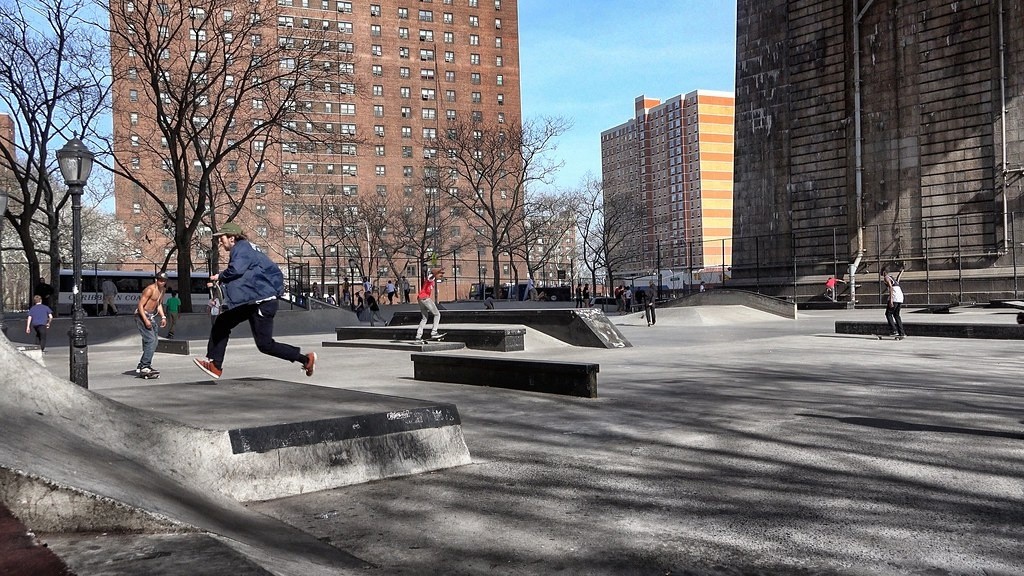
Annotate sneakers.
[193,358,222,379]
[301,351,317,377]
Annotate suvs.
[590,298,627,312]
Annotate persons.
[416,269,445,340]
[825,277,846,301]
[193,223,317,379]
[134,272,168,373]
[100,278,119,316]
[208,290,222,328]
[164,291,181,337]
[299,276,411,327]
[39,277,54,308]
[880,266,906,336]
[575,281,706,327]
[484,296,495,309]
[26,295,53,353]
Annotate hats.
[432,268,445,274]
[156,272,168,279]
[213,222,242,236]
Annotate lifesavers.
[209,279,223,328]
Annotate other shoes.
[115,312,118,316]
[431,332,442,338]
[898,333,906,337]
[416,338,423,342]
[168,331,174,337]
[385,321,389,326]
[136,366,157,374]
[890,330,899,336]
[648,323,651,327]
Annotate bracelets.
[162,317,166,319]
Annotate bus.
[58,268,213,317]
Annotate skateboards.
[824,293,836,302]
[871,333,907,341]
[141,370,160,380]
[416,332,448,344]
[168,332,174,339]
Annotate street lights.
[206,249,214,300]
[349,258,354,308]
[55,130,95,389]
[330,246,340,306]
[482,268,487,300]
